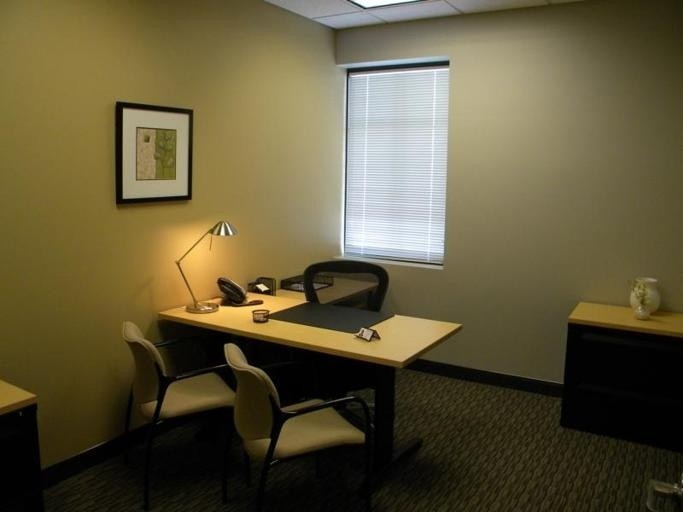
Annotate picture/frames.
[114,102,193,204]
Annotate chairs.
[223,341,373,510]
[301,258,388,314]
[119,319,239,511]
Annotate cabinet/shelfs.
[558,303,683,452]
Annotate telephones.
[217,278,246,303]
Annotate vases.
[629,277,661,316]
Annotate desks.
[157,275,461,493]
